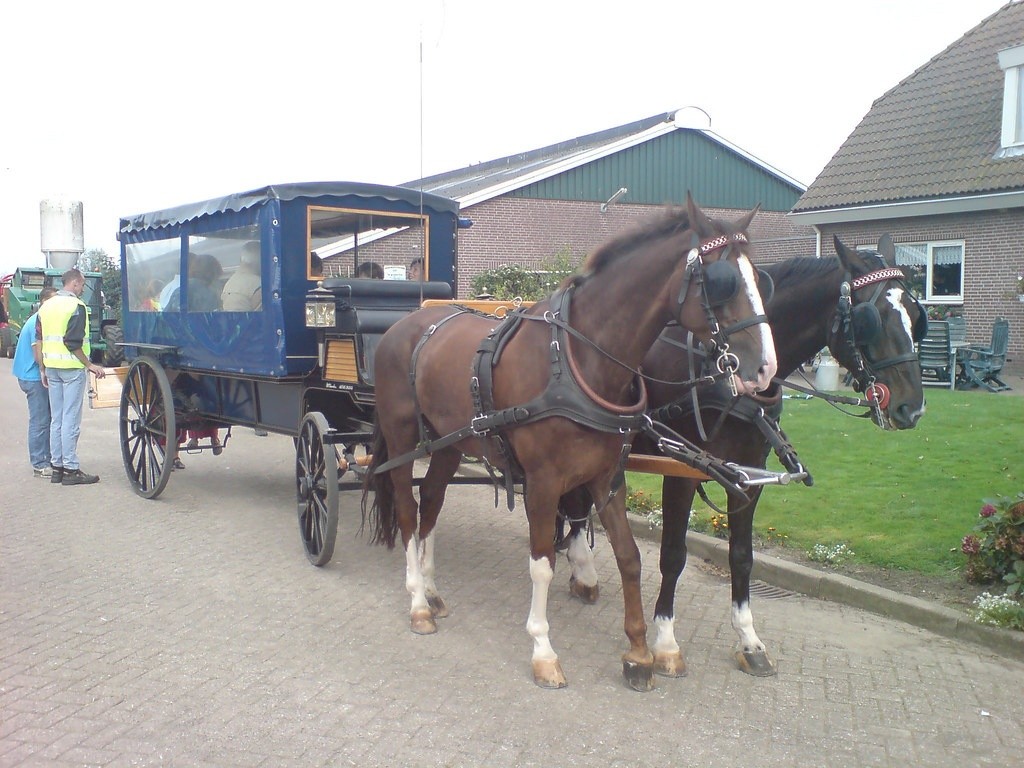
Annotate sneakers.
[62,468,99,486]
[51,465,64,483]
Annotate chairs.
[917,321,957,390]
[956,316,1014,393]
[945,316,967,384]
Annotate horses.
[354,190,778,693]
[552,232,929,679]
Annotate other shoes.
[173,457,185,468]
[188,439,199,448]
[34,465,53,479]
[212,437,222,455]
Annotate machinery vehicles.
[0,266,124,369]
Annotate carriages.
[89,177,931,695]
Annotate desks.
[914,341,971,384]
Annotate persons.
[12,287,59,479]
[35,273,105,485]
[138,239,425,472]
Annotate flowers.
[924,305,962,320]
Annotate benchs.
[324,279,453,389]
